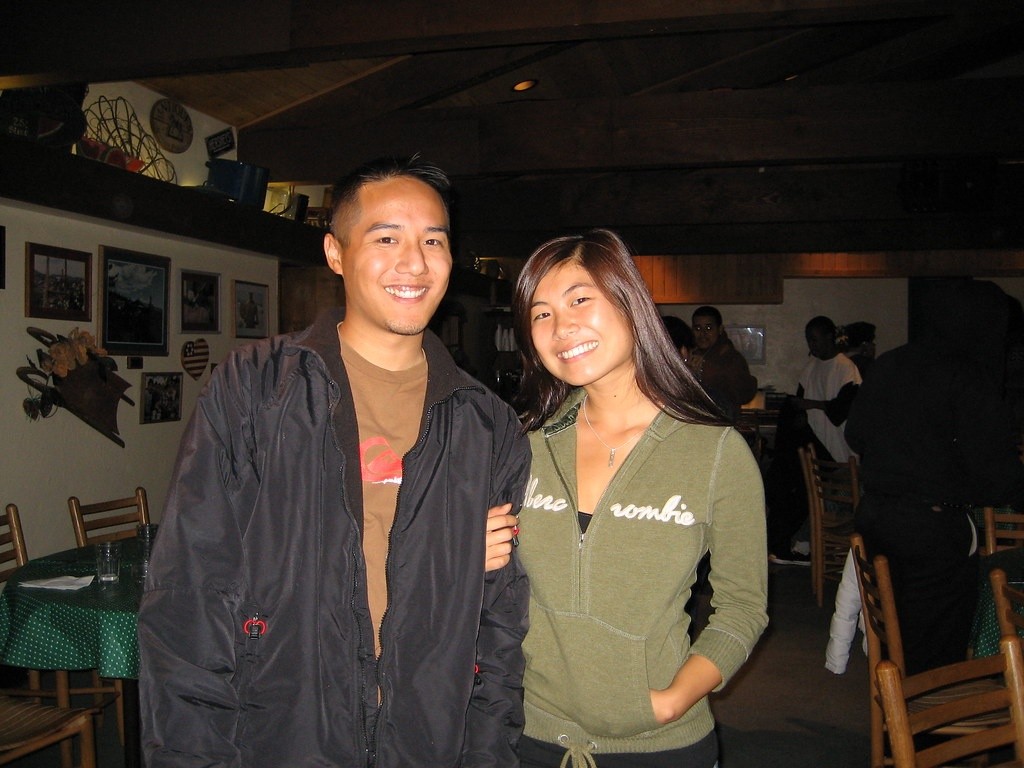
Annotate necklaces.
[584,394,644,468]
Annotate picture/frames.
[724,323,766,365]
[24,241,92,322]
[139,371,182,424]
[232,279,271,338]
[96,244,169,354]
[177,268,222,334]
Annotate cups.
[95,541,126,588]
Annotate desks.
[968,546,1024,657]
[0,534,157,768]
[838,460,1016,531]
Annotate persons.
[768,315,876,566]
[661,306,758,417]
[241,292,259,329]
[844,280,1024,752]
[137,156,533,768]
[145,379,176,420]
[485,229,769,768]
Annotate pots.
[206,156,272,212]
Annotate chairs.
[0,504,56,705]
[797,440,1024,768]
[67,487,150,746]
[0,699,101,768]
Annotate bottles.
[132,523,159,603]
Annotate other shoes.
[768,546,811,566]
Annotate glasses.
[692,323,718,332]
